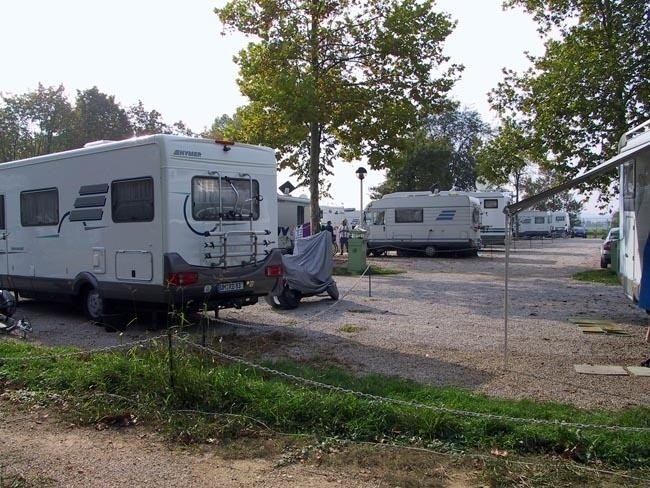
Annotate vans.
[617,119,650,316]
[516,209,571,238]
[352,191,513,257]
[0,134,279,329]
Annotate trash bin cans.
[348,229,367,272]
[611,241,619,272]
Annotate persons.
[337,218,349,255]
[325,220,334,238]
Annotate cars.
[601,227,621,269]
[571,226,587,238]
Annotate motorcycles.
[264,230,339,310]
